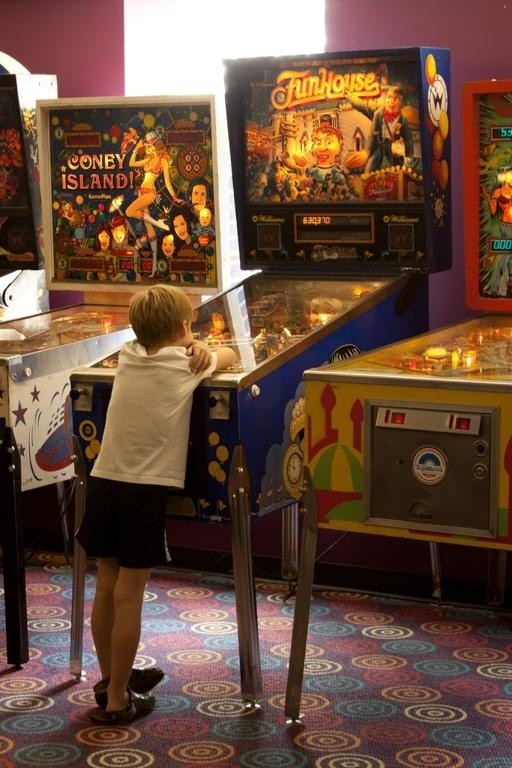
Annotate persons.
[280,124,350,184]
[56,131,214,278]
[73,282,237,724]
[344,86,413,175]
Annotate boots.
[143,213,170,231]
[148,239,159,278]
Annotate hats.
[111,215,125,229]
[143,131,162,146]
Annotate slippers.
[99,688,155,723]
[94,668,163,705]
